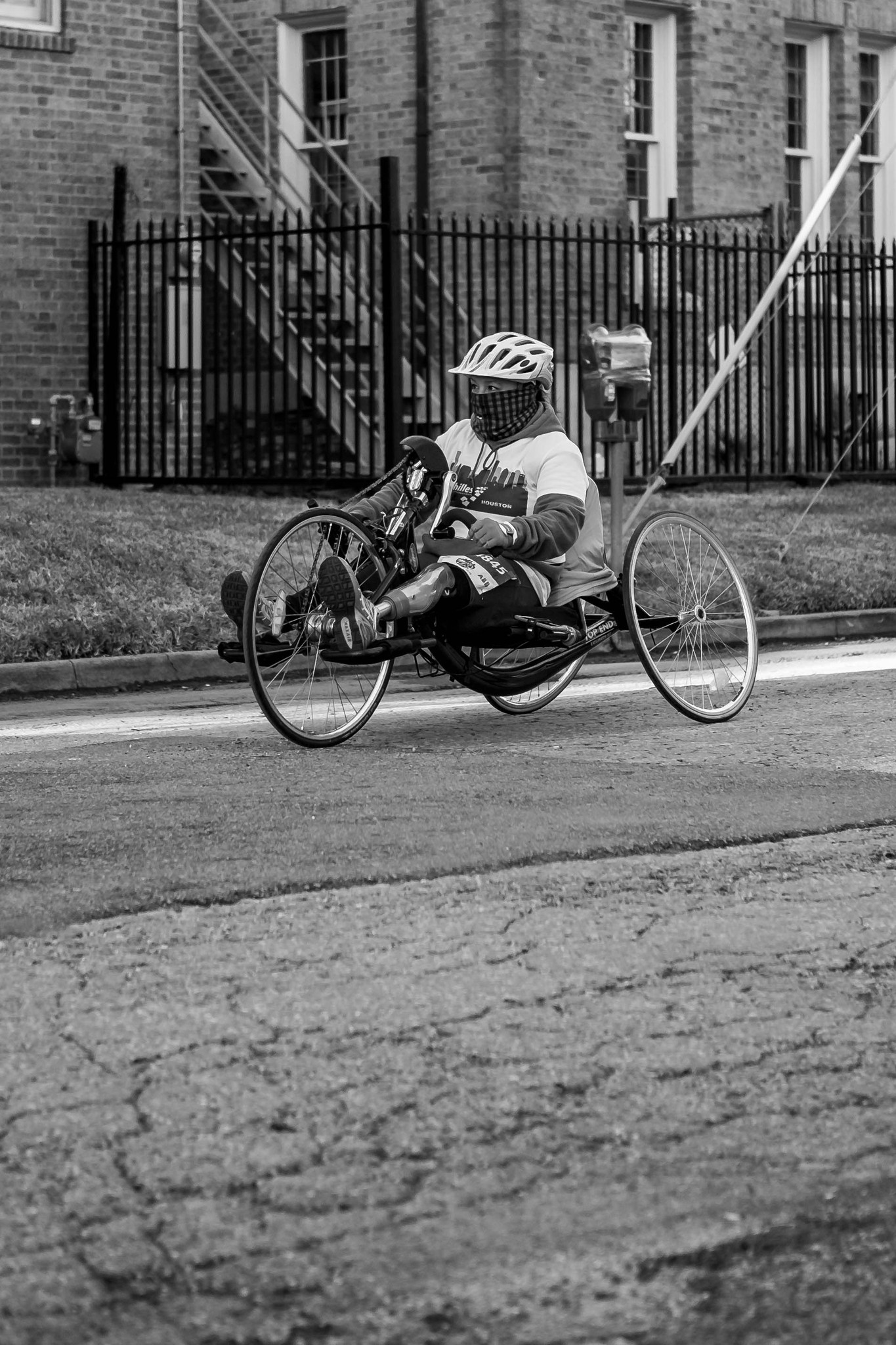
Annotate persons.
[221,331,589,655]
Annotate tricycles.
[217,435,759,748]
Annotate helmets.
[448,332,554,391]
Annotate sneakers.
[220,570,286,643]
[318,556,386,653]
